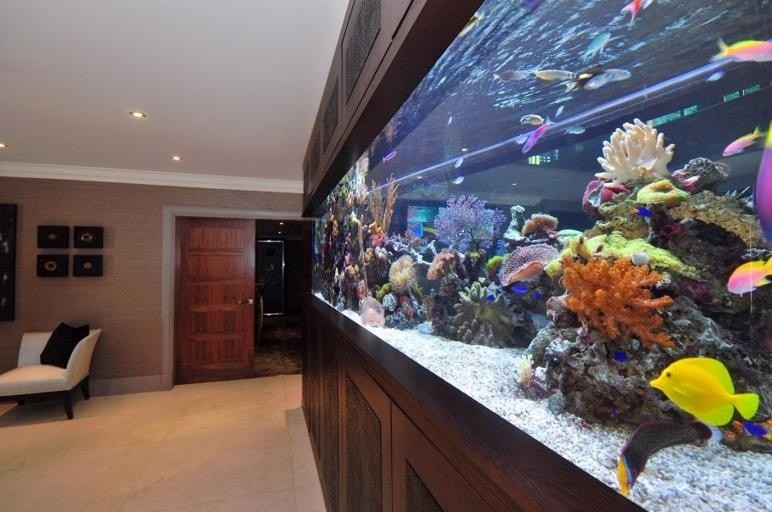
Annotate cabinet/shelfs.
[301,305,495,512]
[301,0,413,210]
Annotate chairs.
[0,328,103,420]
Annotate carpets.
[255,313,304,378]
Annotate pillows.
[54,323,89,367]
[40,322,72,364]
